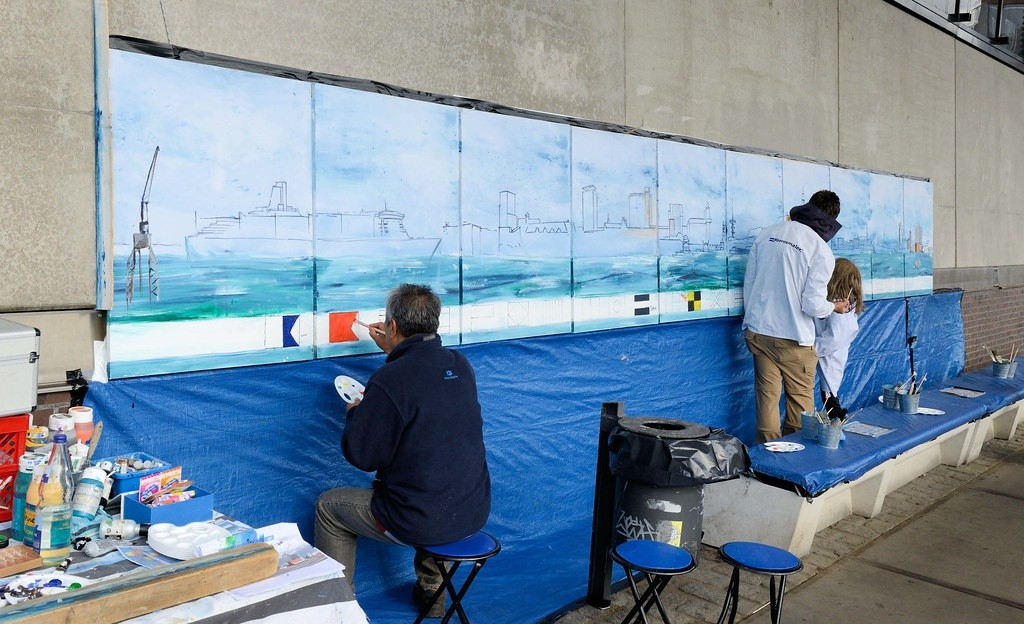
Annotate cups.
[802,411,825,440]
[993,362,1010,379]
[897,389,920,412]
[883,384,903,409]
[816,422,841,449]
[1007,361,1018,378]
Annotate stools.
[610,539,697,624]
[409,533,501,624]
[718,541,804,624]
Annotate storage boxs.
[0,415,34,523]
[0,317,41,417]
[89,450,216,527]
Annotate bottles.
[73,468,107,520]
[23,464,50,546]
[99,519,150,540]
[33,435,74,566]
[26,426,51,455]
[49,413,76,448]
[98,461,114,510]
[68,405,94,447]
[11,453,48,547]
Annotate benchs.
[701,354,1024,557]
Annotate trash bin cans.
[610,418,710,567]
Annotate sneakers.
[412,583,445,618]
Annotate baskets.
[0,471,18,522]
[0,414,29,473]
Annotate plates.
[918,407,945,415]
[764,441,806,452]
[335,375,366,404]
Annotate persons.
[739,191,850,443]
[313,282,491,619]
[812,257,863,422]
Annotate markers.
[843,287,853,309]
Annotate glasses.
[379,310,397,325]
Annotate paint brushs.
[353,318,386,336]
